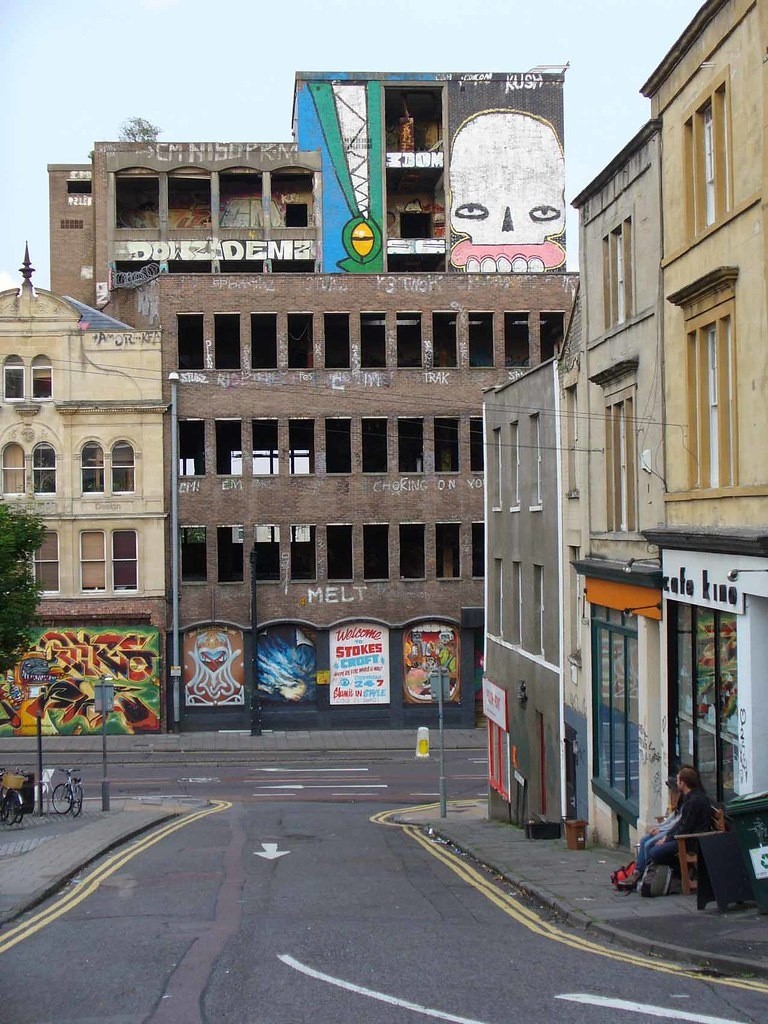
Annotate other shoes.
[617,876,634,888]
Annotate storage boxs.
[523,821,561,839]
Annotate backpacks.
[638,857,672,896]
[610,860,635,890]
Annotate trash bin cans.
[565,819,587,850]
[10,771,35,814]
[724,789,768,915]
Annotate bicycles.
[52,768,84,817]
[0,767,29,826]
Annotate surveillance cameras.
[168,372,179,382]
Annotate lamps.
[517,682,528,702]
[726,568,768,582]
[622,558,660,574]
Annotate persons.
[616,764,713,886]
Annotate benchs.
[654,786,726,895]
